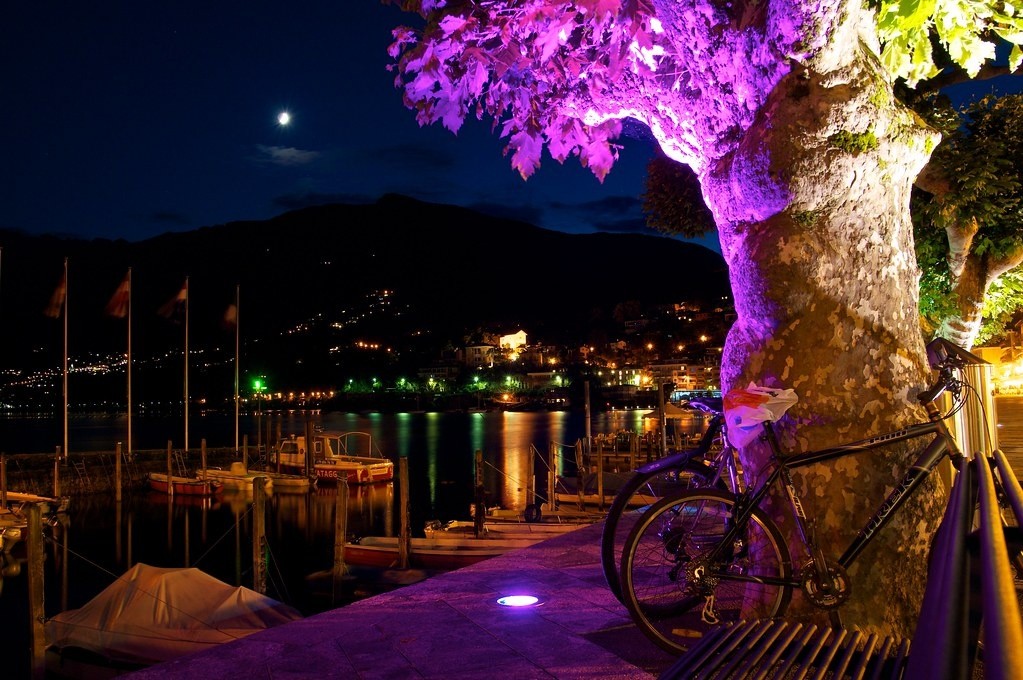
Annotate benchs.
[655,450,1023,680]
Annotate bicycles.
[601,393,752,620]
[619,340,1023,657]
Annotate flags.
[163,277,188,317]
[45,261,67,318]
[223,287,239,329]
[108,267,131,317]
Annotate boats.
[147,471,225,496]
[196,461,310,487]
[345,442,711,569]
[267,428,395,486]
